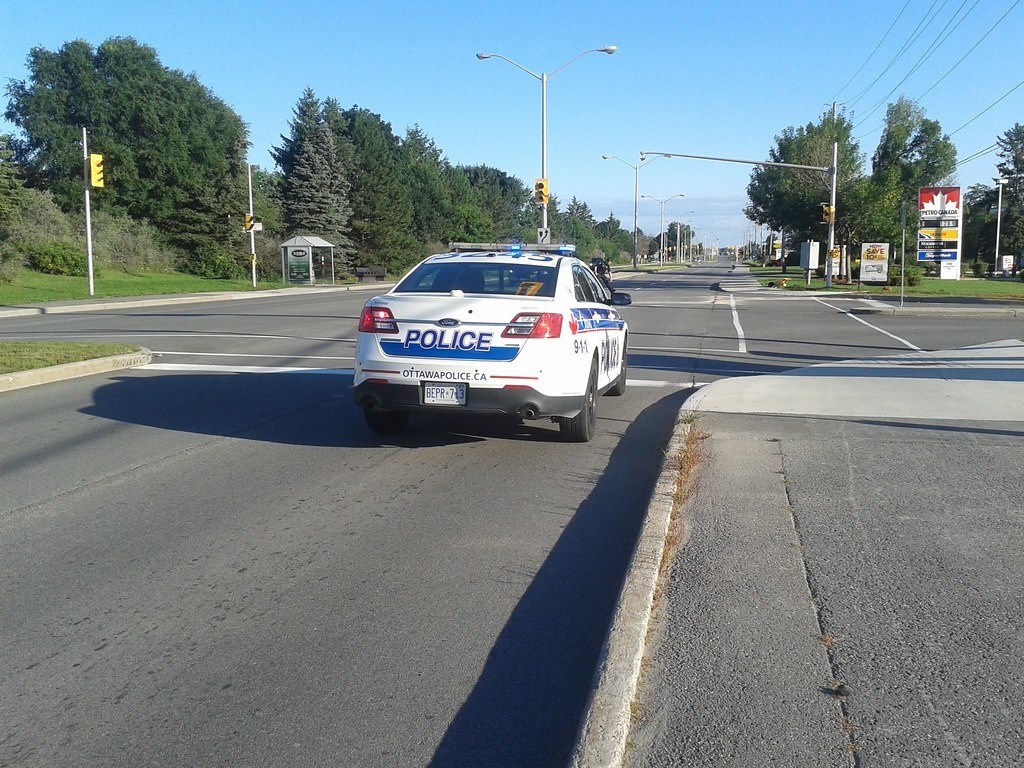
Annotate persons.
[593,256,617,294]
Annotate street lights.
[639,192,774,269]
[475,44,618,244]
[601,153,672,269]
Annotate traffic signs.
[918,229,959,240]
[917,218,958,229]
[917,251,957,261]
[917,239,958,251]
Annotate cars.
[352,242,632,443]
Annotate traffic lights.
[815,205,829,225]
[535,179,548,203]
[245,214,254,233]
[90,153,106,188]
